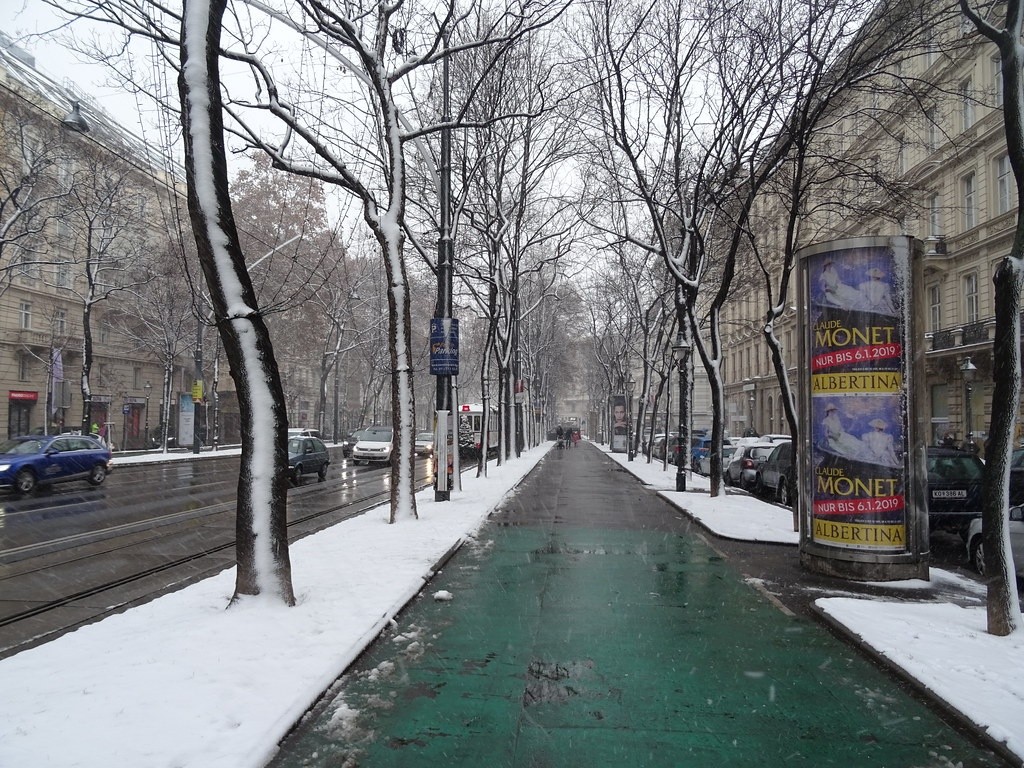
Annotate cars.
[286,426,330,484]
[413,432,435,457]
[901,444,1024,580]
[548,427,581,442]
[632,426,798,515]
[342,425,396,465]
[151,421,206,448]
[0,434,114,494]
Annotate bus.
[458,403,498,448]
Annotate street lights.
[143,380,151,450]
[625,373,636,461]
[958,356,977,452]
[671,330,690,492]
[749,395,756,428]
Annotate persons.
[819,257,896,314]
[557,426,564,445]
[565,428,573,449]
[657,425,665,434]
[572,430,581,446]
[938,431,953,445]
[961,433,980,453]
[743,428,759,438]
[92,421,105,436]
[614,403,627,434]
[822,403,899,465]
